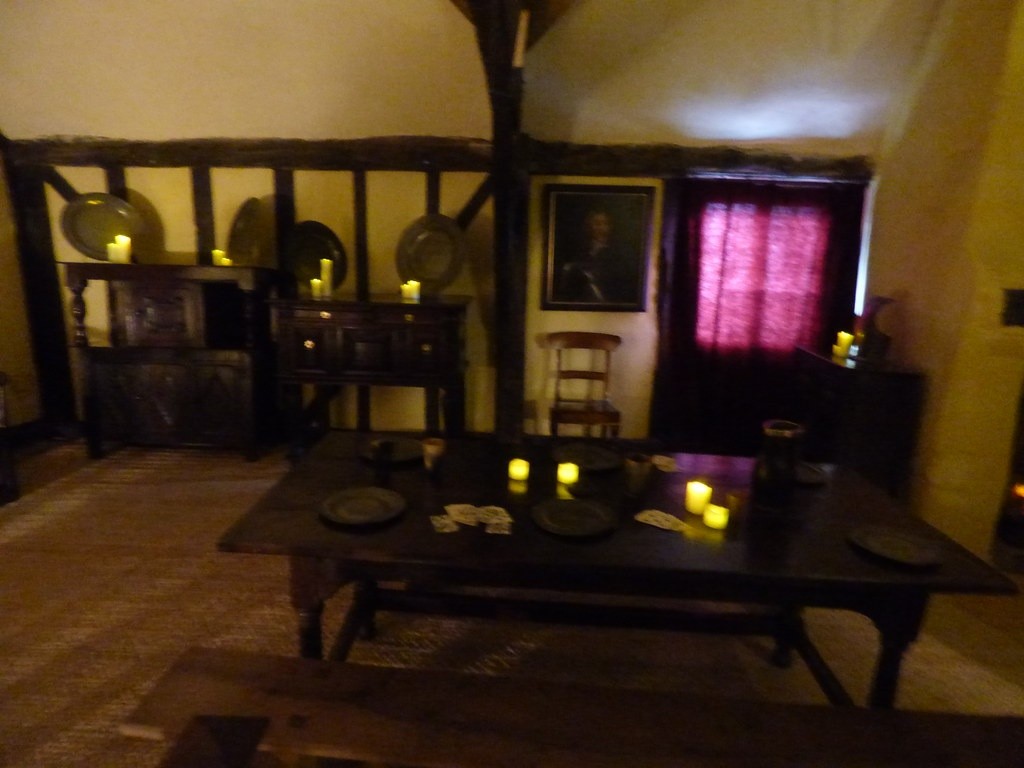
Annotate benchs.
[123,645,1024,768]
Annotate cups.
[422,438,447,469]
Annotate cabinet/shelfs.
[790,348,925,499]
[55,257,278,462]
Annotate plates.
[62,193,143,260]
[295,220,347,290]
[531,498,616,536]
[850,526,946,565]
[316,486,405,523]
[396,214,465,295]
[756,459,829,485]
[553,444,620,470]
[353,438,422,459]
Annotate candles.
[402,278,421,299]
[557,463,581,484]
[106,233,132,262]
[310,257,335,296]
[507,457,530,480]
[212,249,231,265]
[703,507,730,529]
[683,475,712,513]
[836,331,854,357]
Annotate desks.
[214,430,1024,711]
[274,296,471,449]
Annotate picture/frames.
[538,184,656,313]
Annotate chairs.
[536,333,622,437]
